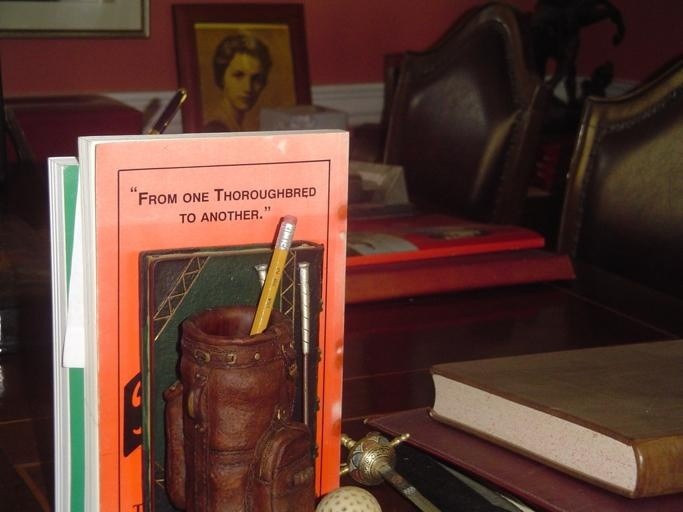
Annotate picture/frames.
[171,3,311,133]
[0,0,150,40]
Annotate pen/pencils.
[149,88,187,135]
[249,214,298,337]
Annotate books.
[427,338,683,499]
[46,127,351,511]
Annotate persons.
[205,33,274,132]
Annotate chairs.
[557,53,683,297]
[350,2,552,230]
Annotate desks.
[0,159,683,511]
